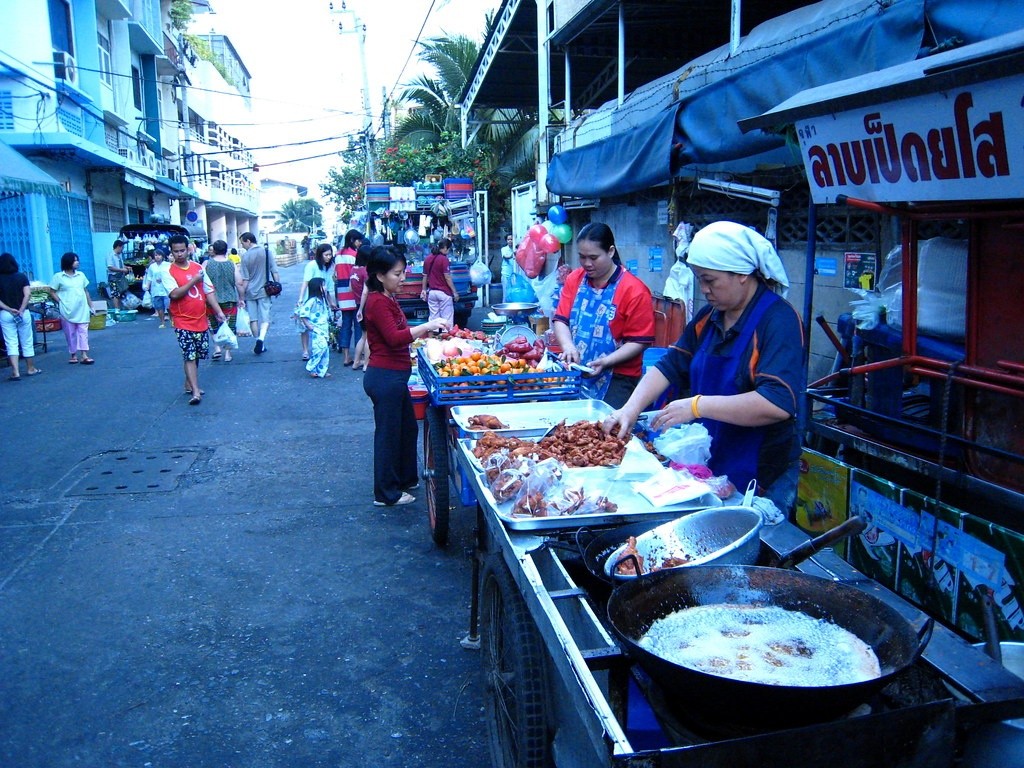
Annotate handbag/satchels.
[141,291,153,308]
[264,281,282,296]
[121,292,142,309]
[214,321,239,349]
[236,306,253,337]
[116,276,129,293]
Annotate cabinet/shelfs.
[0,302,47,367]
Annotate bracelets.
[692,395,702,418]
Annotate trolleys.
[412,323,1024,767]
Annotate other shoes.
[151,312,159,317]
[211,352,222,361]
[225,355,233,362]
[159,325,165,328]
[338,349,342,353]
[164,313,171,321]
[302,353,310,360]
[254,340,267,354]
[324,372,331,377]
[332,342,337,348]
[308,370,318,377]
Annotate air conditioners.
[168,168,180,182]
[147,149,162,176]
[118,148,136,162]
[52,51,78,87]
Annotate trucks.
[349,208,479,330]
[117,223,208,314]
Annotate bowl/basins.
[35,319,62,332]
[115,310,138,322]
[107,309,120,320]
[604,506,764,582]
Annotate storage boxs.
[108,309,137,321]
[417,346,582,405]
[88,315,106,330]
[35,318,62,332]
[91,301,110,314]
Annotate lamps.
[697,177,780,207]
[562,199,600,210]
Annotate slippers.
[80,357,95,363]
[408,483,421,489]
[189,395,201,404]
[69,358,78,363]
[27,367,43,376]
[344,360,353,367]
[186,388,204,395]
[352,362,364,370]
[373,491,416,505]
[8,374,21,381]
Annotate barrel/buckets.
[481,319,506,335]
[941,642,1024,768]
[409,390,428,420]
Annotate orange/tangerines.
[436,352,563,397]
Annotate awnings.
[0,138,62,202]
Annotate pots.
[576,517,868,586]
[608,555,934,725]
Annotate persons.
[363,245,451,505]
[229,248,241,264]
[553,222,656,410]
[162,235,226,403]
[145,249,174,329]
[50,253,96,364]
[300,277,334,378]
[203,240,245,362]
[298,242,335,359]
[603,221,808,519]
[200,245,213,265]
[239,232,281,355]
[106,240,129,308]
[332,242,338,257]
[335,228,363,367]
[0,253,42,381]
[420,238,459,332]
[188,244,200,263]
[501,233,513,302]
[349,244,373,373]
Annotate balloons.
[530,205,572,252]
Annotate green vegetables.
[28,289,50,303]
[123,258,151,265]
